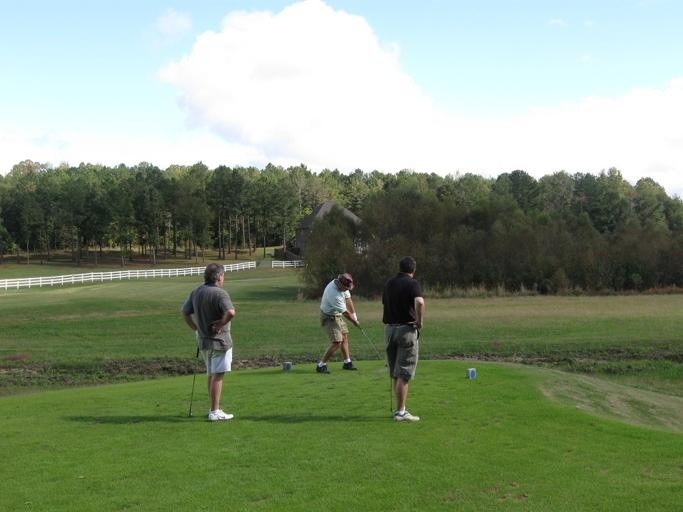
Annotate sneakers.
[393,410,420,422]
[208,409,234,422]
[342,362,357,370]
[316,364,331,374]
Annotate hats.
[337,272,355,291]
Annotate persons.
[315,272,361,373]
[182,263,236,420]
[383,255,425,421]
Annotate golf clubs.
[358,325,389,368]
[187,346,199,416]
[390,377,397,413]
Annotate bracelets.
[351,312,357,320]
